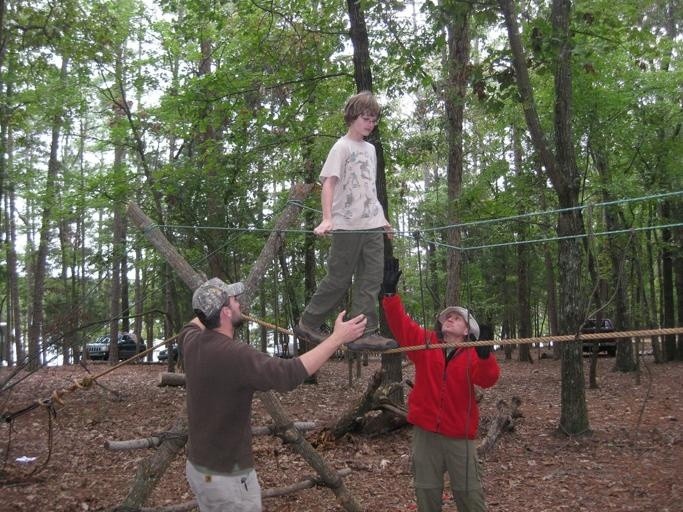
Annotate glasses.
[360,114,379,126]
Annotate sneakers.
[294,317,331,346]
[348,334,398,350]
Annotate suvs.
[87,333,146,359]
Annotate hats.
[437,306,480,340]
[192,278,244,320]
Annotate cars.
[158,346,177,360]
[582,318,617,356]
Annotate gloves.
[470,326,493,358]
[383,257,402,293]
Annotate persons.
[178,277,367,512]
[383,255,501,512]
[292,91,398,350]
